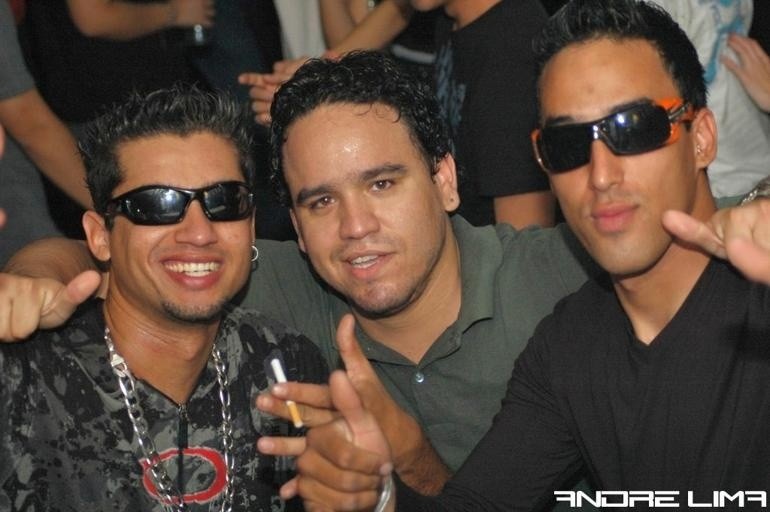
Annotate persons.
[2,0,770,512]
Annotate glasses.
[539,101,690,174]
[106,180,260,226]
